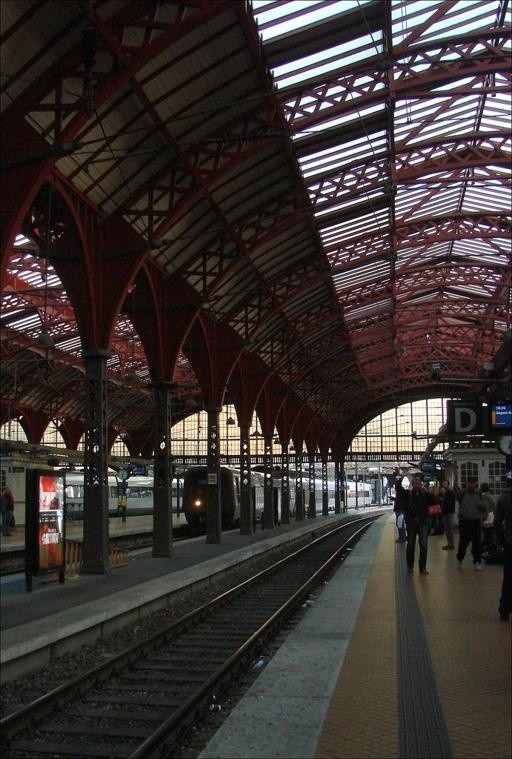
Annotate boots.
[394,528,406,543]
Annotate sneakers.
[472,562,482,571]
[456,556,464,569]
[442,545,454,549]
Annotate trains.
[182,463,373,532]
[64,471,185,519]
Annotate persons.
[389,478,497,575]
[493,471,511,622]
[1,487,14,536]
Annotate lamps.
[185,345,198,408]
[290,425,294,450]
[252,392,261,437]
[124,274,141,386]
[34,184,58,351]
[273,410,280,445]
[226,378,235,425]
